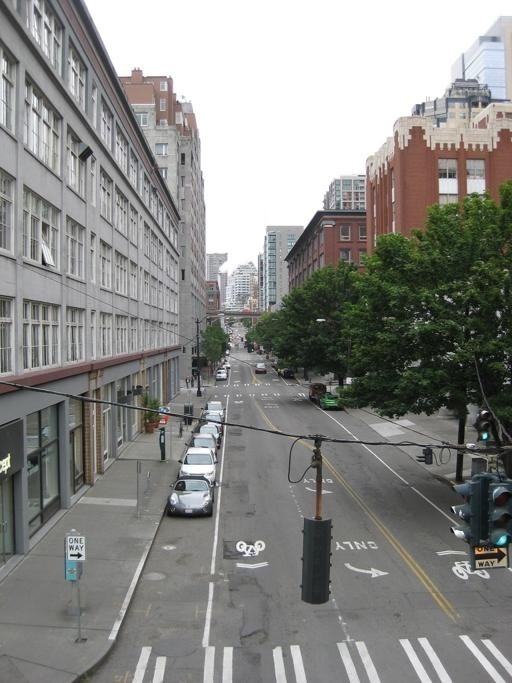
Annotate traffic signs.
[66,536,86,561]
[471,547,509,569]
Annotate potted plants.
[150,400,160,429]
[144,398,155,434]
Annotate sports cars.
[167,475,216,517]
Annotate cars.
[177,446,218,482]
[309,383,344,410]
[216,360,230,381]
[184,433,219,452]
[195,400,226,444]
[255,362,267,374]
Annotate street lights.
[316,318,352,367]
[195,312,225,397]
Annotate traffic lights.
[451,473,512,547]
[475,409,491,441]
